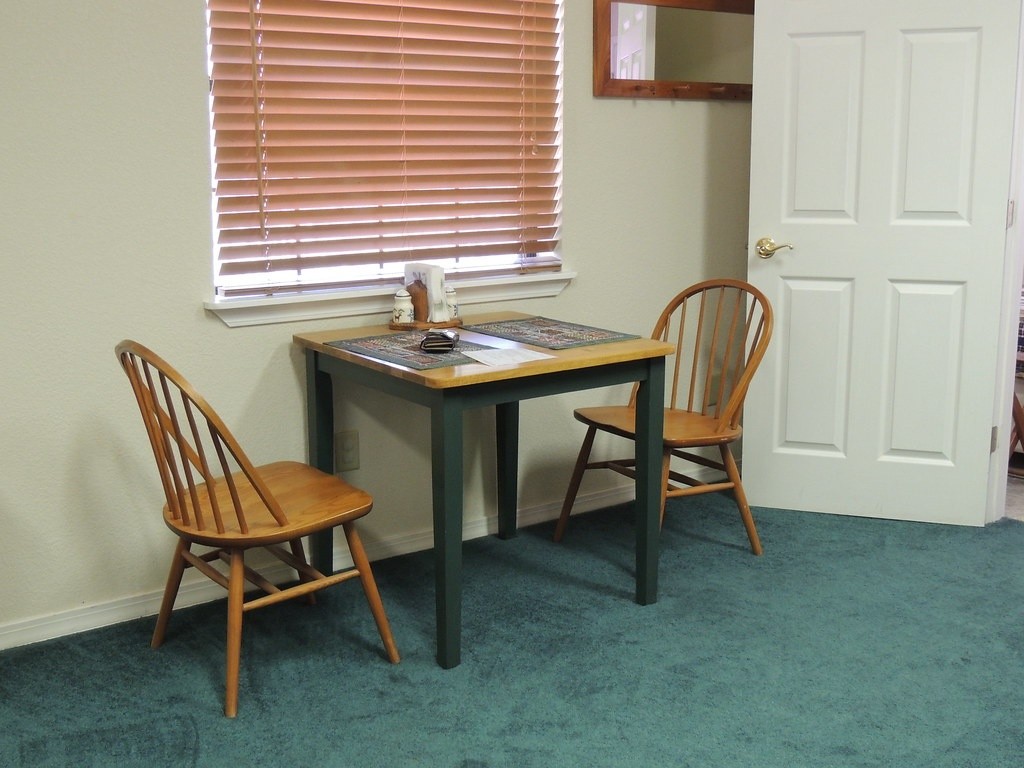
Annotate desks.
[295,311,678,667]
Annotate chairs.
[115,338,401,718]
[554,279,773,556]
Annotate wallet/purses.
[420,327,459,351]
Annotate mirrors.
[591,0,755,101]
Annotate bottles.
[445,285,458,320]
[391,290,414,323]
